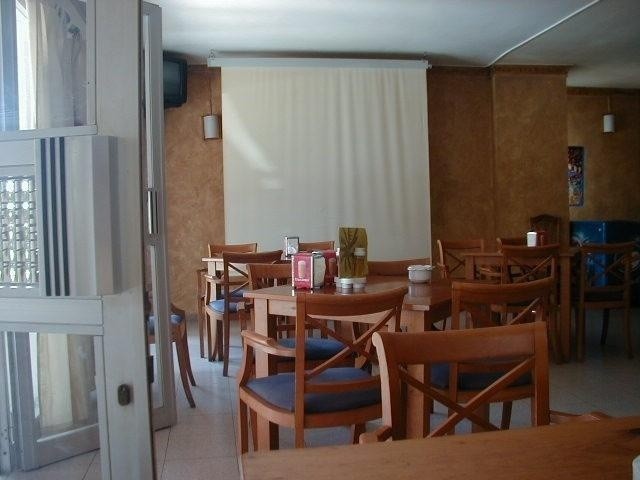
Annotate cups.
[526,231,537,247]
[406,264,433,284]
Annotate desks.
[243,274,502,452]
[242,416,640,480]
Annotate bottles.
[311,250,327,289]
[298,260,306,278]
[329,258,337,274]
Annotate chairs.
[195,214,564,409]
[358,320,601,445]
[571,239,636,360]
[426,276,551,437]
[145,283,194,409]
[238,285,409,452]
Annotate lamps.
[601,88,616,136]
[200,67,221,141]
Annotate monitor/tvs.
[163,56,188,107]
